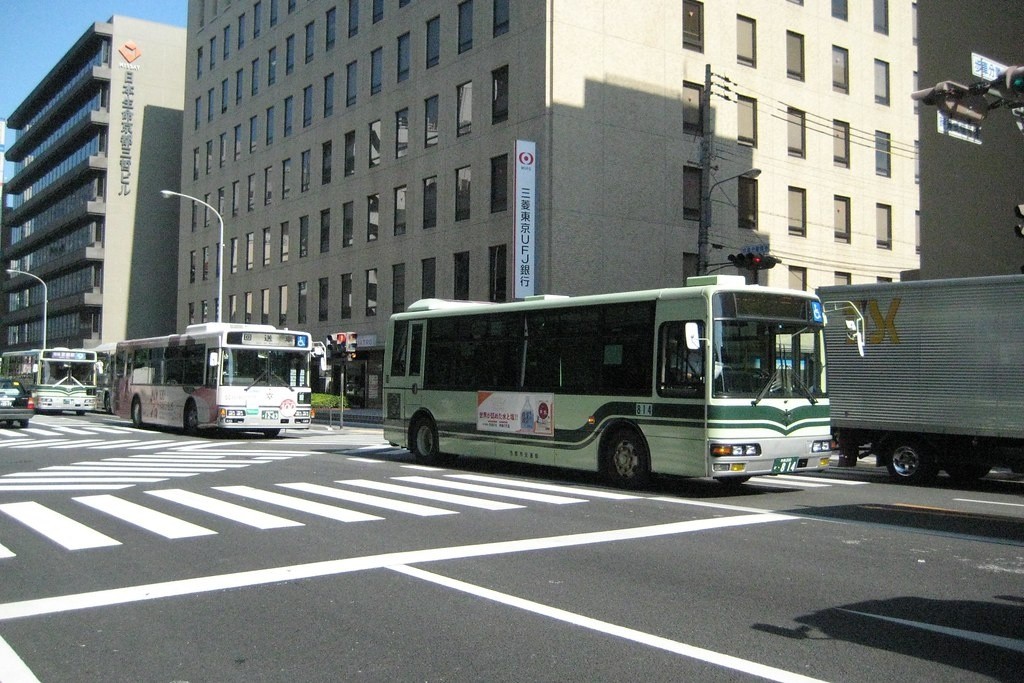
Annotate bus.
[113,322,327,438]
[0,347,97,415]
[382,274,866,492]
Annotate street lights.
[6,269,47,349]
[697,169,761,275]
[160,190,224,323]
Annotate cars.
[0,379,35,427]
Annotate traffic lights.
[747,256,783,270]
[346,332,358,361]
[911,83,986,123]
[327,334,337,359]
[336,333,346,359]
[727,254,764,267]
